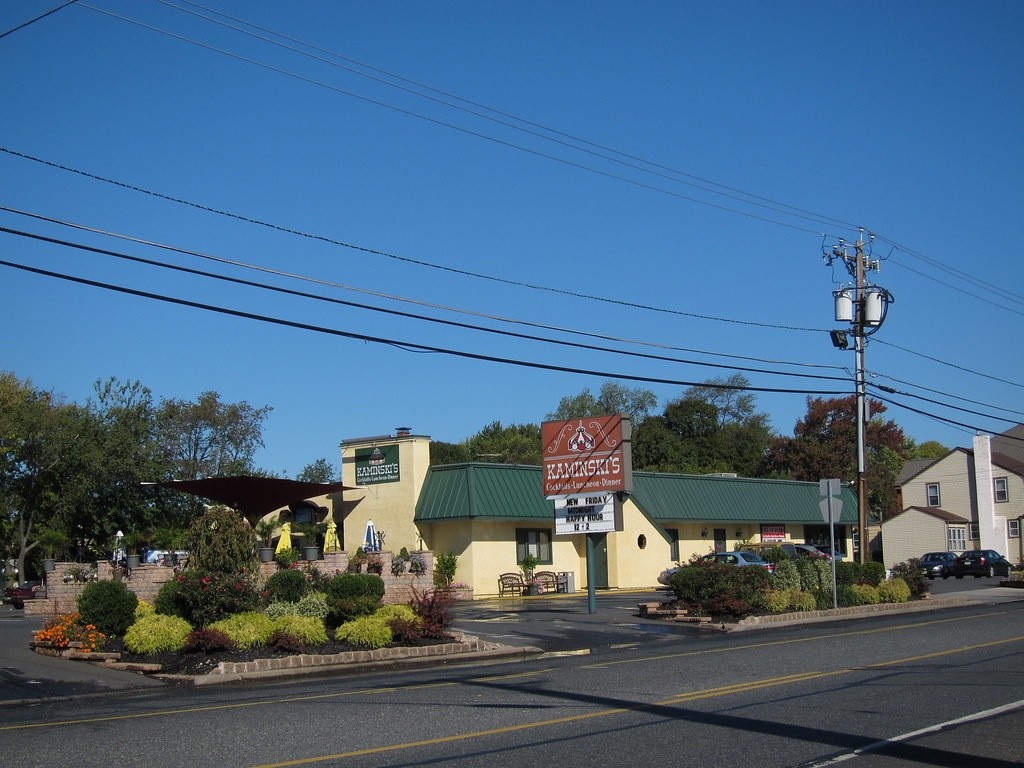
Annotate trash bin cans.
[558,571,575,594]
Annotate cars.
[954,550,1012,580]
[917,552,961,580]
[656,551,775,581]
[2,578,46,610]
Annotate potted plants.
[433,552,458,602]
[123,523,148,568]
[148,528,191,567]
[521,554,542,595]
[31,566,48,598]
[104,535,135,582]
[347,554,362,573]
[66,567,89,582]
[287,521,328,561]
[253,516,283,562]
[398,547,412,573]
[38,527,71,572]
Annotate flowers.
[368,554,386,570]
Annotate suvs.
[794,544,843,565]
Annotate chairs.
[535,571,558,593]
[499,573,525,597]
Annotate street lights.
[822,225,893,571]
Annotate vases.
[368,565,378,573]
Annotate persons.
[112,531,127,569]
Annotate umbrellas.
[363,519,379,552]
[275,522,292,555]
[324,519,340,553]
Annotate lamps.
[736,527,744,538]
[702,526,709,538]
